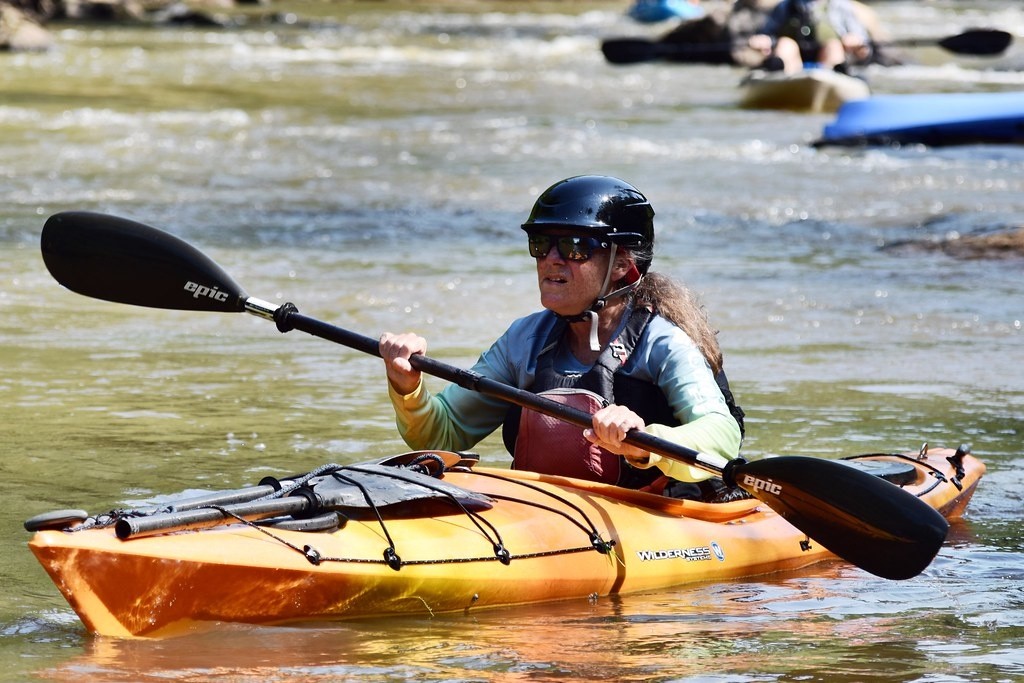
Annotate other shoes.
[763,56,784,73]
[833,63,847,75]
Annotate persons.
[747,0,873,75]
[379,174,741,502]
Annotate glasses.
[527,231,622,261]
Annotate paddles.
[35,208,955,586]
[597,29,1014,67]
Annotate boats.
[23,446,987,639]
[600,15,750,66]
[737,64,871,113]
[807,91,1024,149]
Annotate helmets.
[522,173,654,253]
